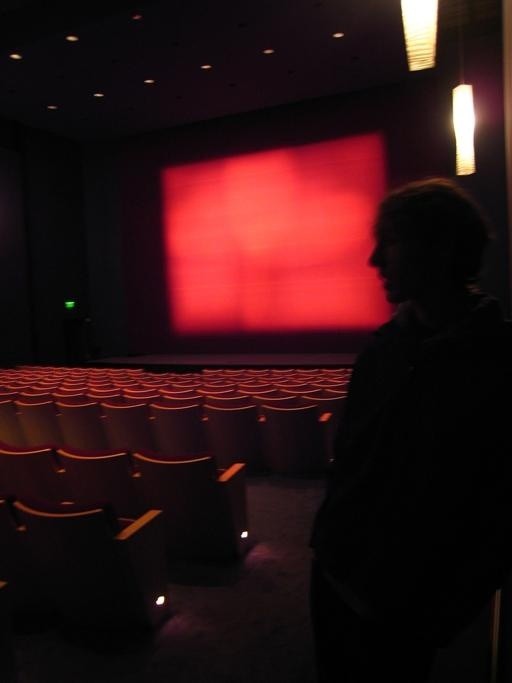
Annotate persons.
[308,177,507,681]
